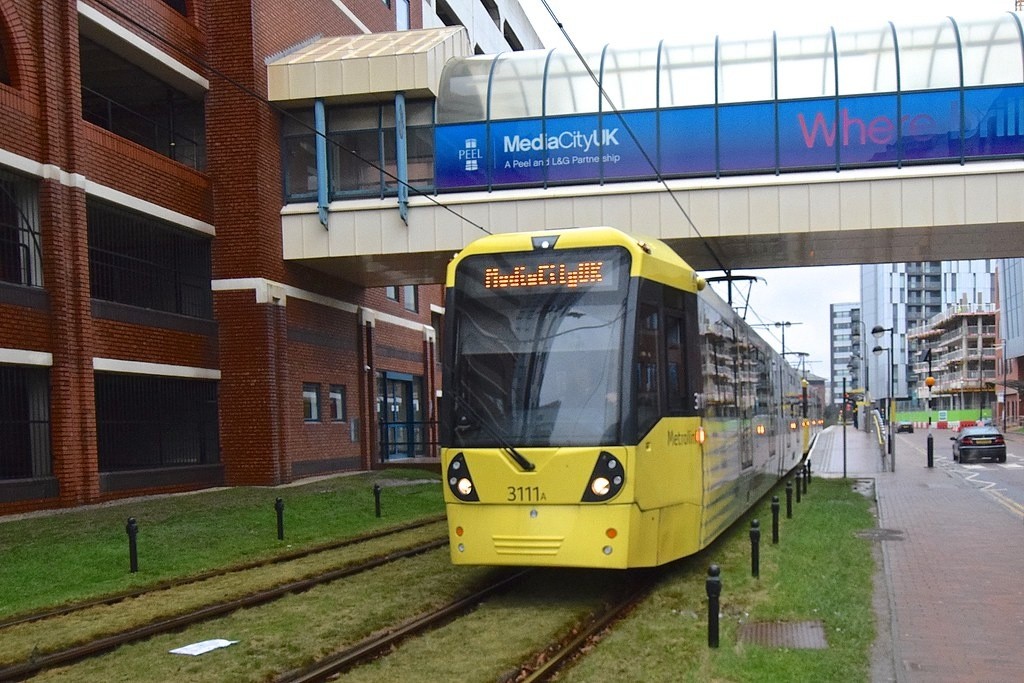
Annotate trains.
[438,226,823,571]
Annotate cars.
[896,421,913,436]
[950,426,1007,463]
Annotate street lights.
[871,347,890,419]
[870,323,892,459]
[924,377,936,469]
